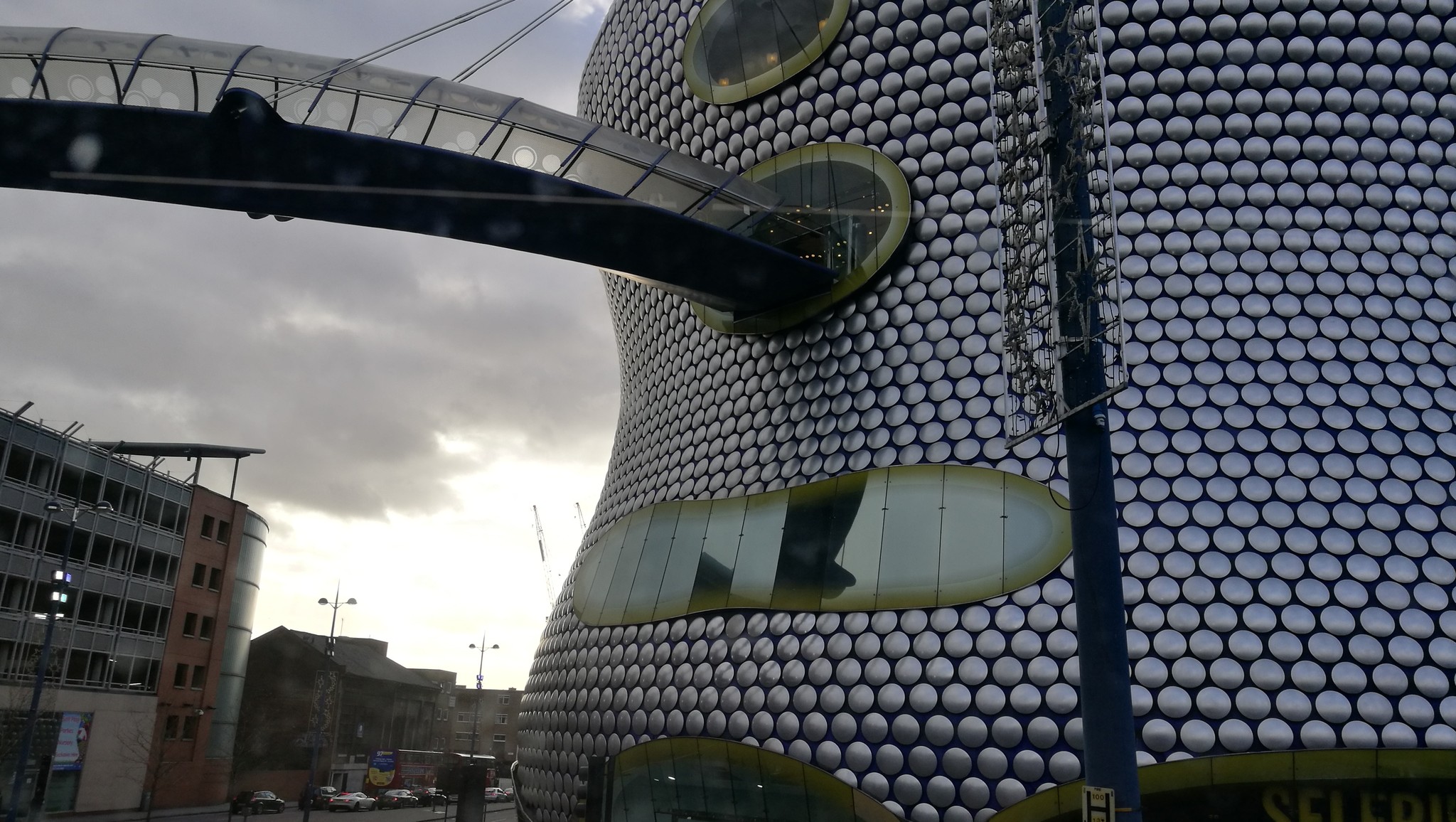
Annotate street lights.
[4,500,115,822]
[468,632,499,785]
[302,580,357,822]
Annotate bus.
[362,747,496,796]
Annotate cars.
[409,787,459,807]
[485,787,508,803]
[375,789,419,810]
[503,788,514,801]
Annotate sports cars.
[329,792,376,812]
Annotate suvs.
[310,786,338,810]
[230,791,285,815]
